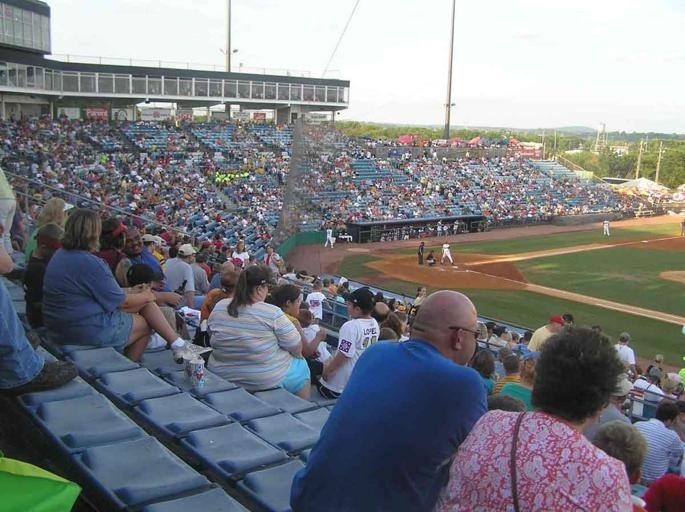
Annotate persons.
[642,473,685,512]
[232,240,248,269]
[10,201,28,251]
[125,264,181,350]
[283,265,426,325]
[378,327,398,340]
[313,287,380,399]
[0,167,78,396]
[263,244,284,279]
[290,290,488,512]
[305,282,332,326]
[94,217,134,290]
[632,401,685,488]
[114,119,404,252]
[471,351,496,394]
[403,137,685,242]
[590,419,649,512]
[562,313,685,404]
[200,254,211,277]
[190,256,210,293]
[40,207,212,365]
[580,371,634,446]
[380,311,409,342]
[213,246,228,274]
[498,357,538,412]
[160,243,198,310]
[433,325,632,511]
[205,266,311,405]
[139,232,165,269]
[25,197,67,267]
[191,270,241,369]
[0,113,113,211]
[123,227,201,328]
[527,313,566,352]
[264,283,326,384]
[207,260,235,290]
[491,356,522,397]
[486,394,527,413]
[244,256,258,271]
[475,319,531,352]
[22,222,66,330]
[231,258,242,274]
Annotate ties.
[266,254,271,265]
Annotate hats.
[610,373,633,397]
[341,288,377,306]
[249,278,278,286]
[101,218,127,240]
[655,355,664,361]
[563,314,574,323]
[649,367,662,378]
[63,202,74,212]
[141,233,158,244]
[551,316,565,324]
[178,244,196,256]
[202,241,210,247]
[126,264,162,283]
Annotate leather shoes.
[0,359,79,397]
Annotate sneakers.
[171,339,214,365]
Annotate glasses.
[501,331,510,334]
[448,327,481,340]
